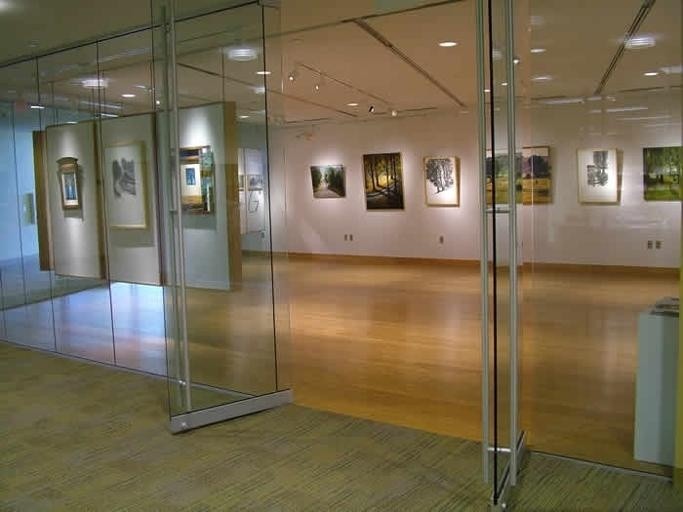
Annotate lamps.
[385,104,398,117]
[288,67,322,95]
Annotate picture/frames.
[54,156,84,211]
[576,146,619,204]
[167,143,216,215]
[424,155,460,208]
[485,144,553,206]
[98,141,152,232]
